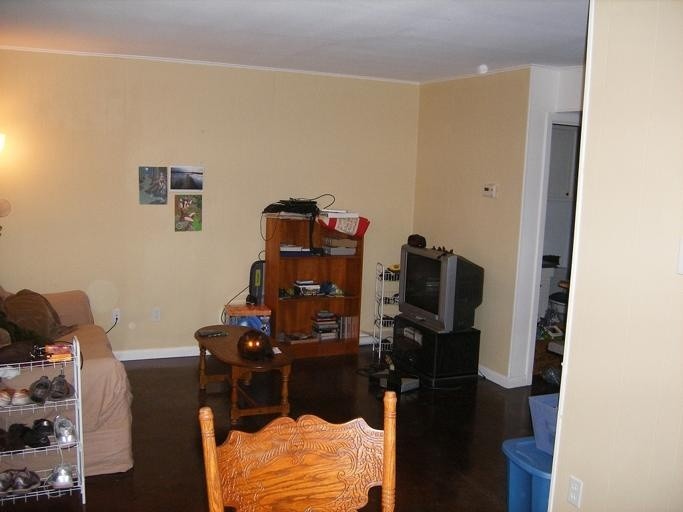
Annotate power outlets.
[112,308,120,323]
[566,475,582,509]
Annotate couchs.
[0,282,138,476]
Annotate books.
[311,310,359,341]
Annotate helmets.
[237,329,274,360]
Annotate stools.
[223,305,270,338]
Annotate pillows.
[4,289,77,338]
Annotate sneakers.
[0,375,79,489]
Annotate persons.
[178,199,195,222]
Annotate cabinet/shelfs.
[372,257,403,360]
[0,334,89,507]
[262,214,365,362]
[391,316,482,387]
[549,123,577,201]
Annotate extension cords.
[384,355,394,371]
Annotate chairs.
[192,388,398,511]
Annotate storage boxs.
[498,436,553,512]
[528,393,560,457]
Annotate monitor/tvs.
[399,244,484,335]
